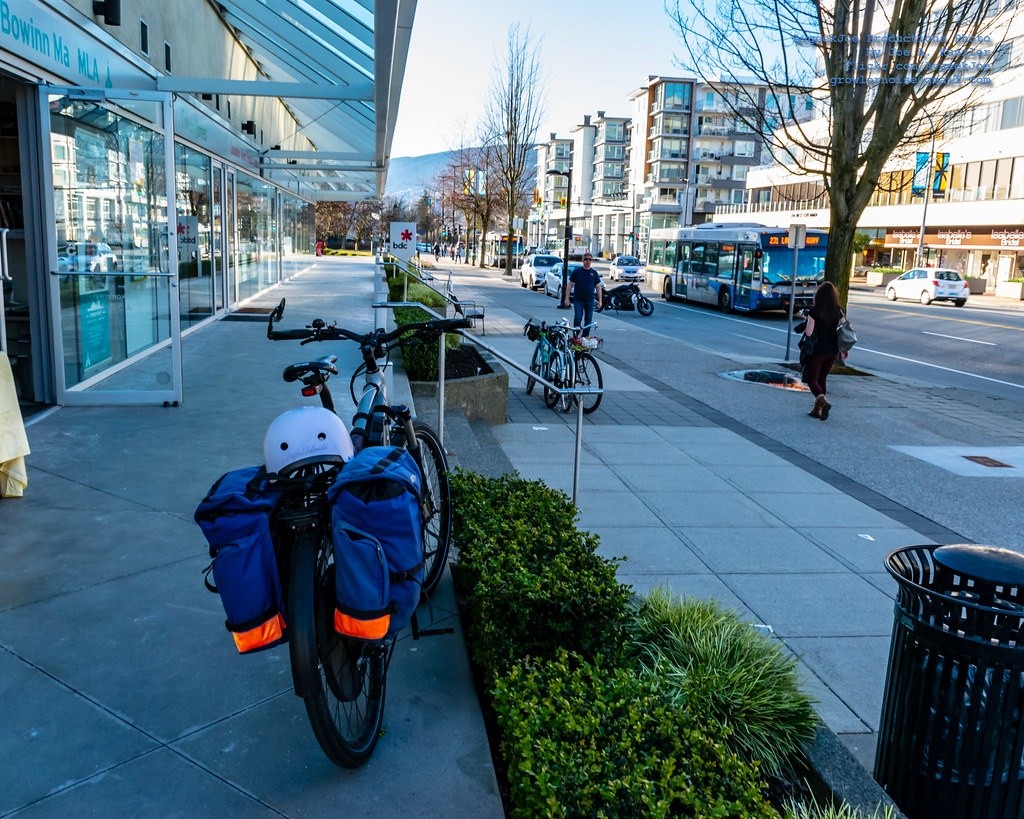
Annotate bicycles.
[524,317,607,415]
[264,295,487,771]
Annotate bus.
[647,221,830,318]
[478,231,525,268]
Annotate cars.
[460,241,465,249]
[717,269,752,279]
[884,268,970,307]
[609,256,645,281]
[59,242,121,283]
[524,246,551,255]
[545,262,607,303]
[416,243,432,252]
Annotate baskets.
[574,336,603,351]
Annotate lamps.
[92,0,121,26]
[270,144,280,150]
[242,121,254,134]
[289,160,297,164]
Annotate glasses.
[583,257,592,261]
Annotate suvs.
[520,253,564,293]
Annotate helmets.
[262,406,354,485]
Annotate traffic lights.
[561,198,566,208]
[454,228,457,234]
[442,225,446,236]
[448,227,450,234]
[459,225,463,234]
[537,197,542,207]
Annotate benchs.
[417,269,434,287]
[446,291,485,336]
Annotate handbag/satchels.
[193,465,290,655]
[836,320,857,352]
[326,446,424,645]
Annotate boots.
[817,396,832,421]
[807,401,822,419]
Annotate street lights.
[546,169,573,310]
[634,225,651,266]
[472,131,514,268]
[680,178,690,228]
[440,164,471,259]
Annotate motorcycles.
[593,278,654,316]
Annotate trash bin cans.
[874,541,1024,819]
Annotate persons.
[450,244,455,260]
[454,245,462,265]
[982,259,995,286]
[800,282,848,421]
[443,243,447,257]
[564,253,602,337]
[434,241,439,262]
